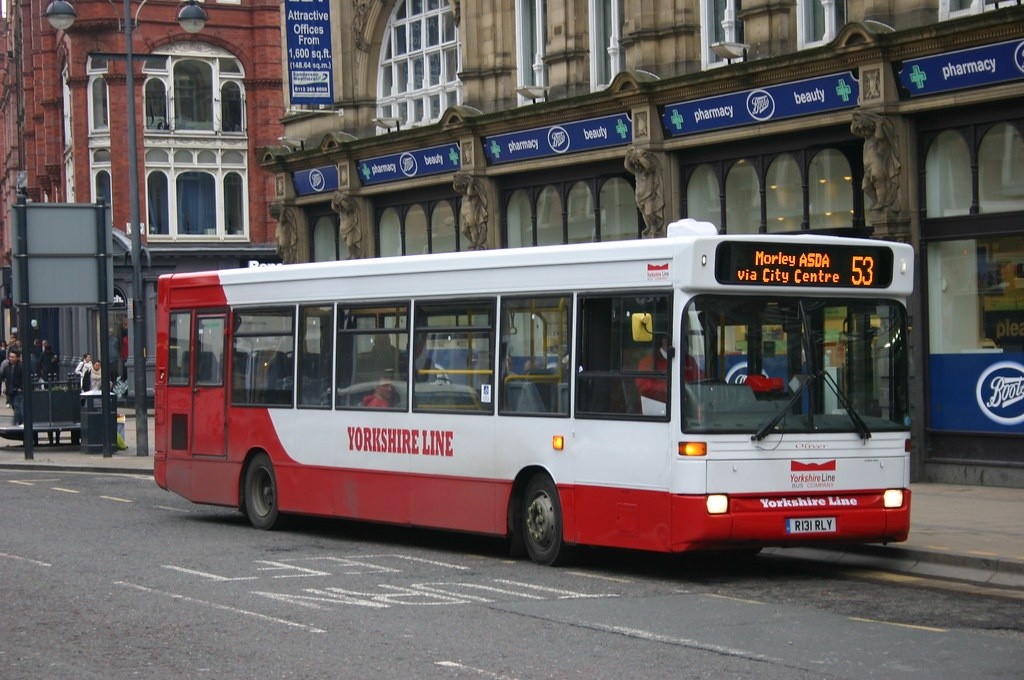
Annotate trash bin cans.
[79,388,118,455]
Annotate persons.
[0,349,24,425]
[330,192,358,259]
[450,171,479,249]
[0,333,57,407]
[849,114,891,212]
[268,202,292,265]
[622,147,657,236]
[108,328,121,383]
[76,353,93,406]
[83,361,102,391]
[637,326,707,401]
[119,319,128,381]
[354,333,407,408]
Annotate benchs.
[0,424,82,447]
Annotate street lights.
[41,2,209,458]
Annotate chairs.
[621,346,652,414]
[182,348,485,414]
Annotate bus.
[155,218,916,567]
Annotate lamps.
[515,86,551,104]
[711,41,750,66]
[371,118,402,134]
[277,136,307,153]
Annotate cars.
[324,379,481,413]
